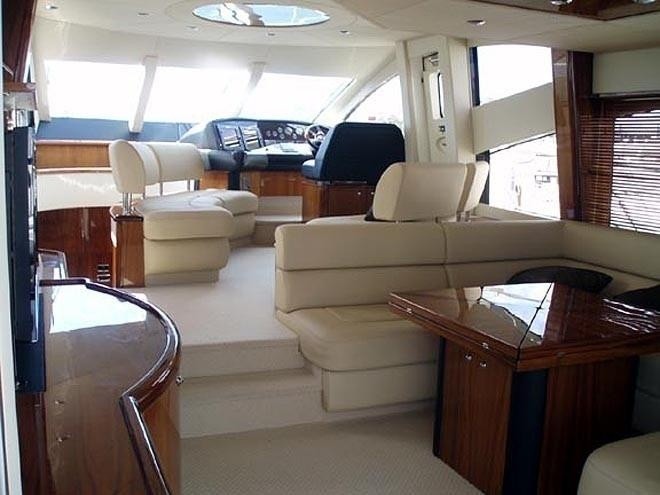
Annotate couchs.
[276,220,659,414]
[575,432,659,494]
[107,138,259,284]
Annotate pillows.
[614,284,659,312]
[505,266,613,294]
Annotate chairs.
[310,160,491,220]
[302,121,406,182]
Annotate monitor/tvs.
[5,124,47,395]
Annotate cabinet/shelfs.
[16,278,180,495]
[302,182,375,222]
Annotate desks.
[387,283,659,495]
